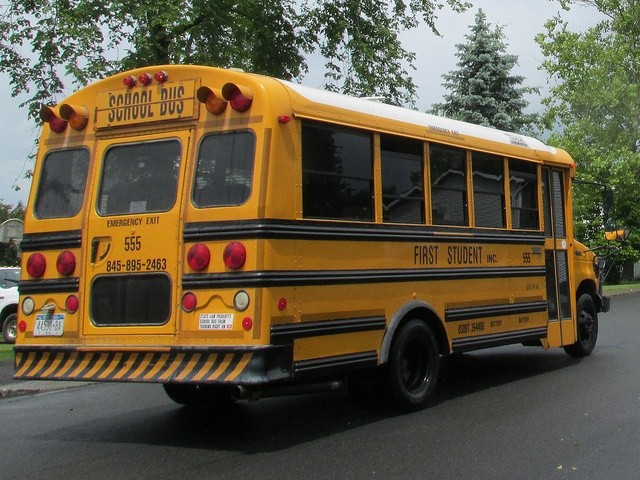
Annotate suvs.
[0,265,25,343]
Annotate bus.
[12,64,630,410]
